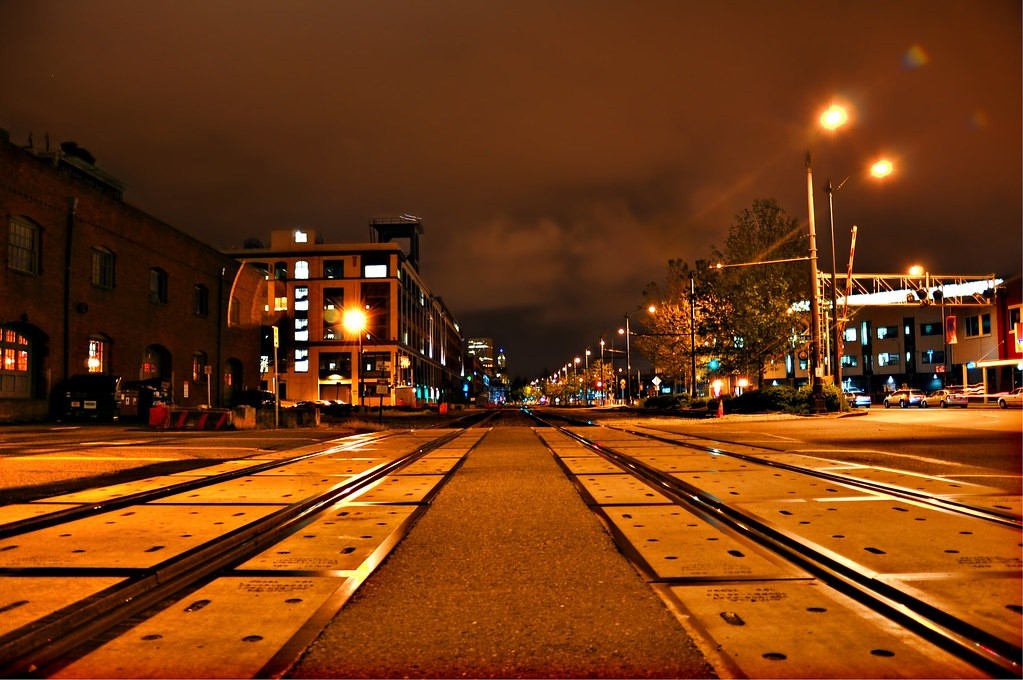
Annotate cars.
[844,387,863,394]
[120,379,175,418]
[882,389,926,408]
[920,390,968,408]
[845,391,872,408]
[49,373,122,424]
[290,398,352,420]
[997,387,1023,409]
[241,389,280,409]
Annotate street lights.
[546,356,581,406]
[824,154,899,397]
[689,268,698,399]
[624,306,657,406]
[585,336,605,400]
[342,306,368,411]
[802,94,854,414]
[599,328,625,406]
[908,265,950,387]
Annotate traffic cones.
[715,398,724,418]
